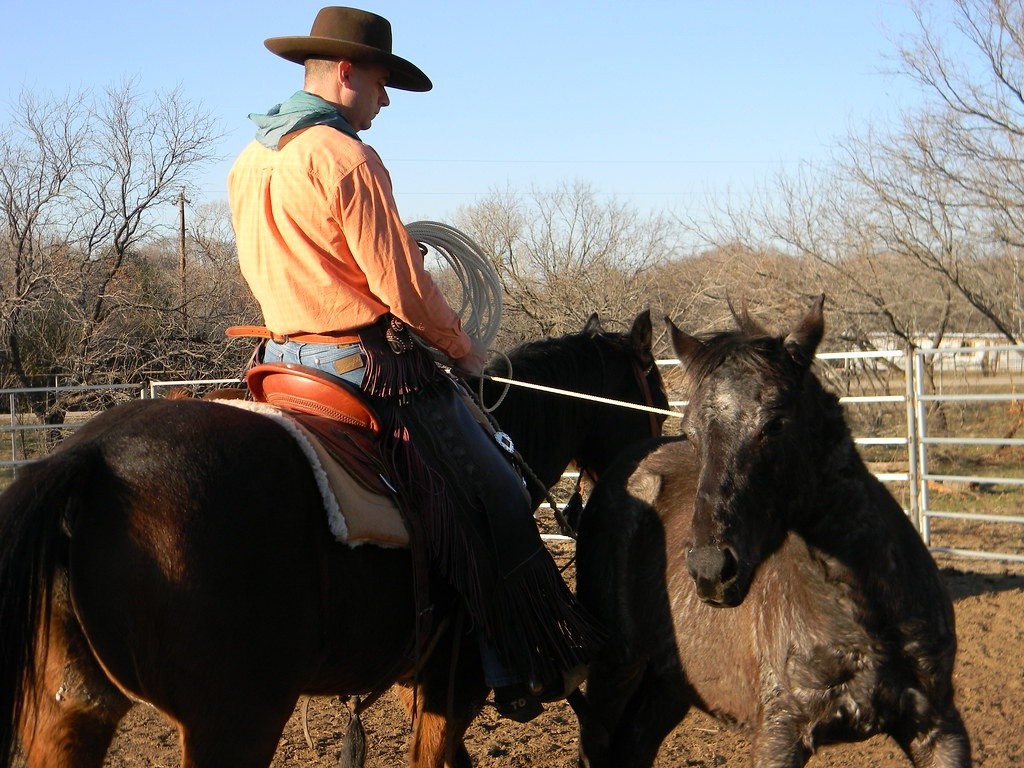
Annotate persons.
[227,6,603,703]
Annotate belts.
[225,325,360,345]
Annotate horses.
[0,303,672,768]
[574,291,973,768]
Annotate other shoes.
[496,654,589,722]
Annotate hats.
[264,6,433,92]
